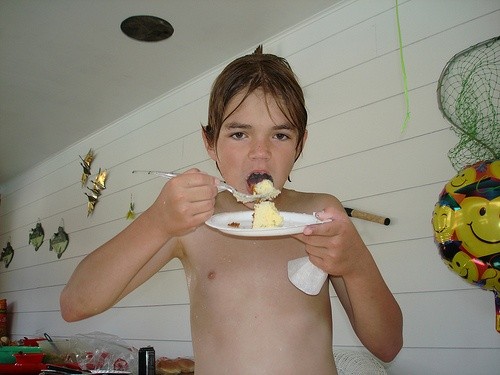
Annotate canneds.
[137,345,156,375]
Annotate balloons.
[432,158,500,335]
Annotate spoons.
[132,169,281,204]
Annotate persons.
[60,45,403,375]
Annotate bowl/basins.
[40,340,70,365]
[13,352,46,363]
[0,345,40,363]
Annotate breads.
[156,358,195,375]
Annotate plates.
[205,210,330,237]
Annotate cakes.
[251,179,284,228]
